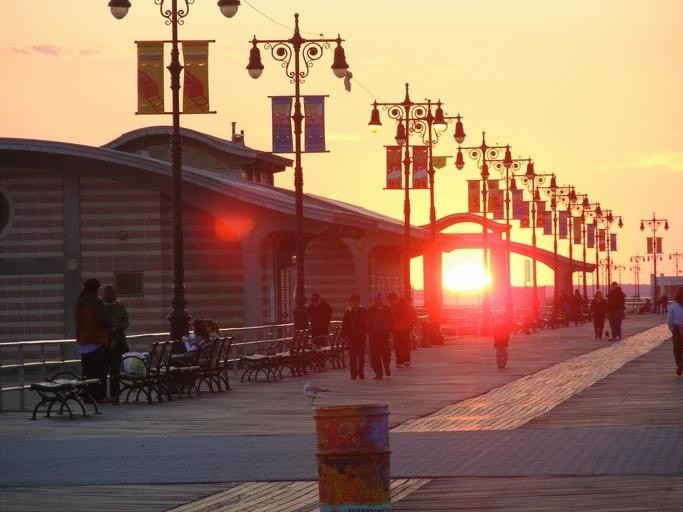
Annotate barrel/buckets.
[312,402,394,512]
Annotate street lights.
[106,1,241,354]
[244,12,351,340]
[365,84,447,344]
[638,213,669,313]
[573,251,683,307]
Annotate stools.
[31,371,103,419]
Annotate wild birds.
[302,380,329,404]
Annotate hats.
[311,293,320,301]
[83,279,101,288]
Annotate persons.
[559,282,626,342]
[161,319,210,368]
[638,294,668,315]
[307,292,333,373]
[205,319,220,370]
[340,295,369,380]
[74,278,110,404]
[667,286,683,376]
[494,316,510,369]
[367,291,392,379]
[388,292,411,368]
[101,285,129,406]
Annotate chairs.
[240,330,345,382]
[116,337,235,403]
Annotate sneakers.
[350,361,411,380]
[83,396,120,405]
